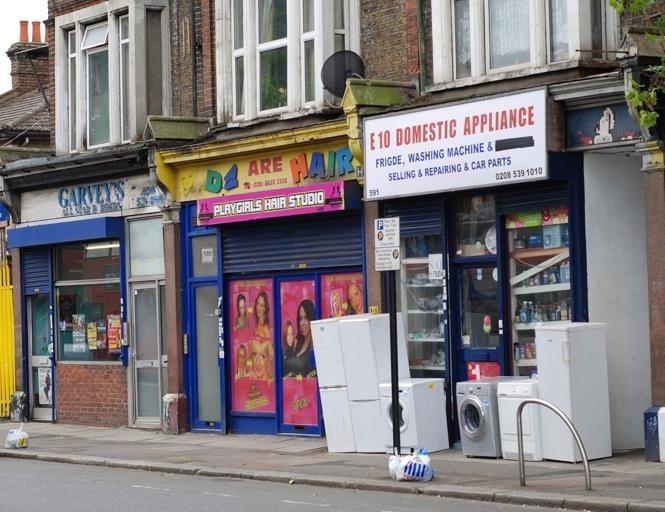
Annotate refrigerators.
[310,312,410,454]
[535,322,614,463]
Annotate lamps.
[84,238,121,250]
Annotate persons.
[283,319,296,375]
[248,340,274,387]
[327,291,347,318]
[235,293,250,329]
[253,291,271,339]
[347,283,365,314]
[290,300,317,378]
[235,342,248,377]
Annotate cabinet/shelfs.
[507,220,575,380]
[394,232,449,372]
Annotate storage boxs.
[458,242,491,259]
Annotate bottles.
[518,340,537,361]
[411,449,430,464]
[515,262,572,320]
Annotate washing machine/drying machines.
[378,378,450,453]
[455,379,502,460]
[496,378,544,461]
